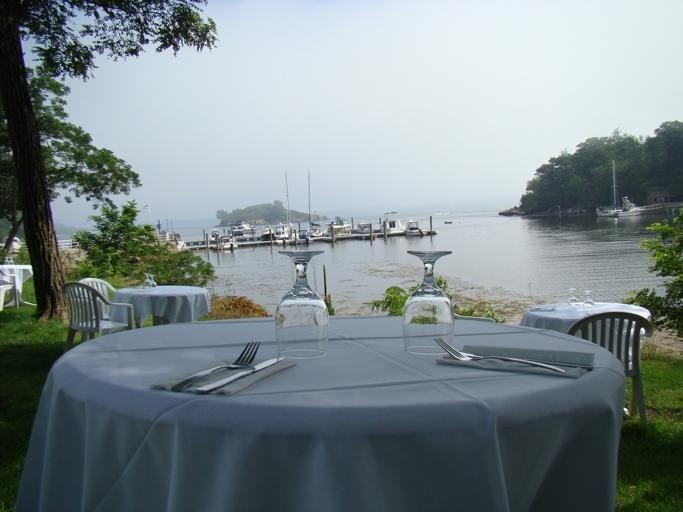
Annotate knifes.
[194,355,284,395]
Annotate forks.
[170,340,259,392]
[431,336,565,374]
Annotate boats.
[144,209,438,254]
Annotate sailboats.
[592,155,645,217]
[301,167,332,243]
[281,170,305,245]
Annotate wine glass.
[3,256,13,266]
[566,288,594,314]
[273,250,329,360]
[148,275,156,289]
[402,250,454,357]
[143,273,151,291]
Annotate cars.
[0,236,21,250]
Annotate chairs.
[564,311,653,425]
[75,278,117,343]
[61,282,136,347]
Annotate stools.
[0,283,14,314]
